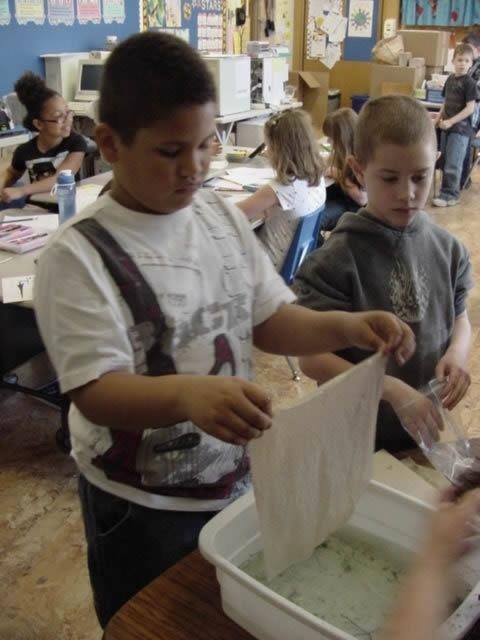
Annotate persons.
[212,108,367,273]
[427,36,480,207]
[377,470,480,640]
[31,30,417,634]
[297,95,473,453]
[0,74,92,206]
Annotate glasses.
[40,109,75,124]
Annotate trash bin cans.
[327,93,341,112]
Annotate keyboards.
[67,102,92,116]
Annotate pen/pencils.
[201,176,244,191]
[1,218,34,223]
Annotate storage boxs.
[395,29,449,67]
[290,59,427,133]
[198,480,480,640]
[426,67,444,81]
[369,62,426,99]
[288,72,329,136]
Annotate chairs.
[280,204,326,286]
[434,151,442,198]
[462,138,480,190]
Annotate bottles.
[50,169,76,226]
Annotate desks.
[103,438,480,639]
[40,101,306,136]
[1,136,335,452]
[214,102,303,143]
[235,116,271,148]
[419,101,444,109]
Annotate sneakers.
[432,198,457,206]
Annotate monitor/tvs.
[73,59,105,101]
